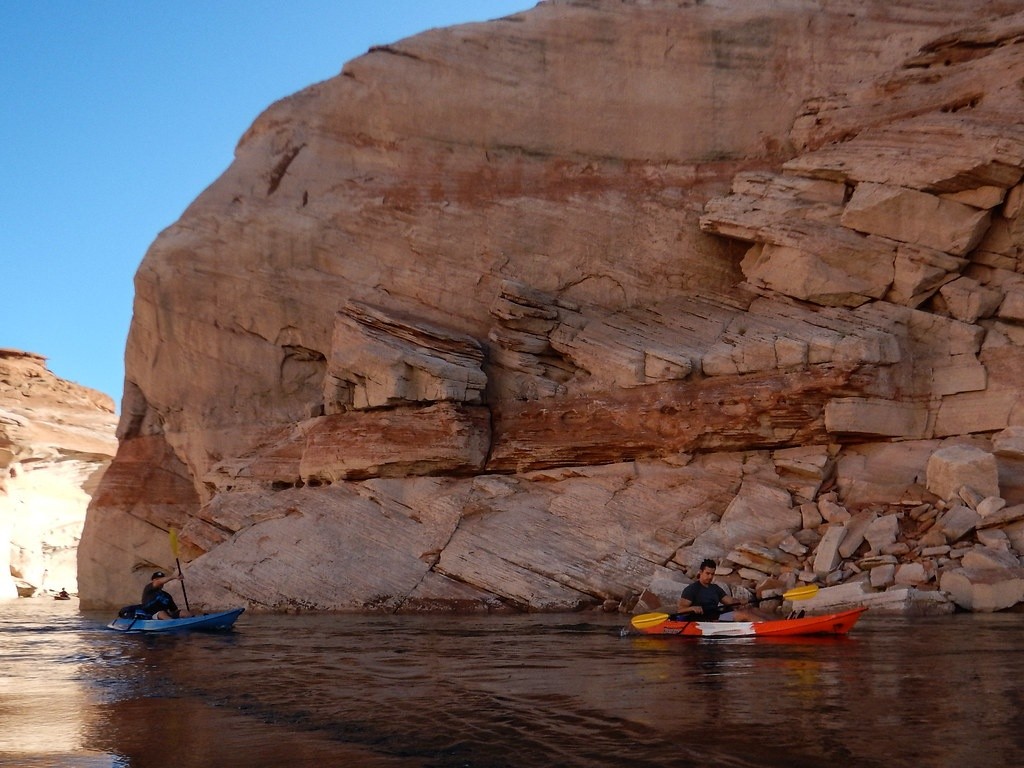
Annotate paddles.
[630,585,819,630]
[168,527,191,613]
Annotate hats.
[151,572,166,580]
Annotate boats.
[54,596,70,600]
[636,607,867,638]
[107,607,245,634]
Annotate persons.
[140,571,209,620]
[59,587,69,597]
[675,558,805,622]
